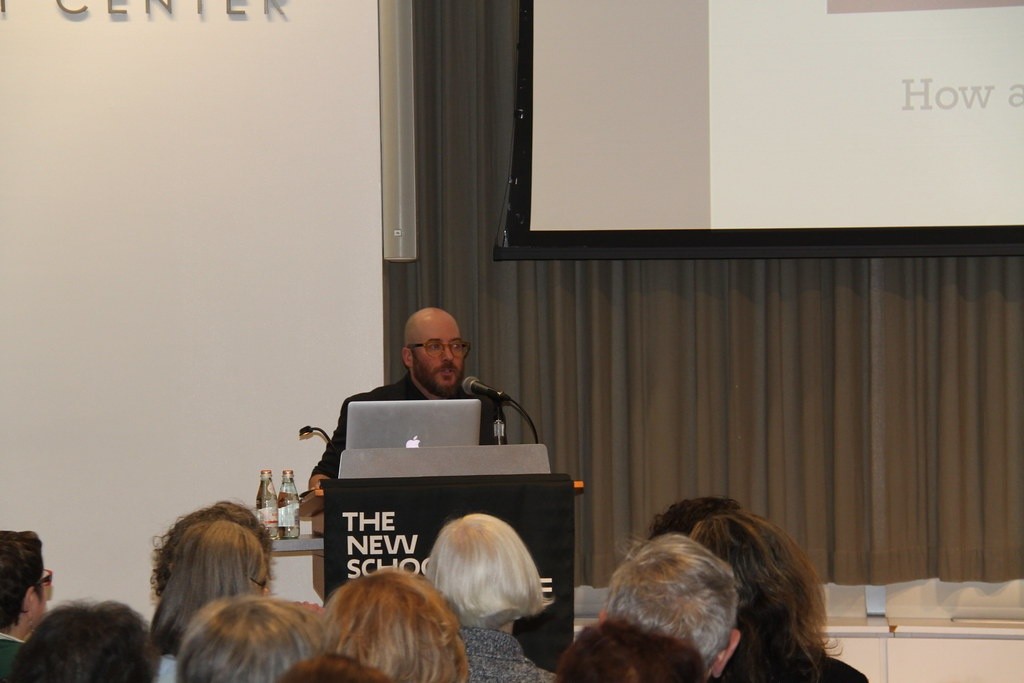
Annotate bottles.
[278,470,300,539]
[256,470,278,540]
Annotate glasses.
[35,569,52,587]
[245,574,266,592]
[406,340,471,360]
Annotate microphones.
[462,376,511,401]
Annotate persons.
[309,308,507,495]
[177,568,467,683]
[152,502,273,683]
[0,530,47,683]
[7,600,160,683]
[423,513,558,683]
[557,496,869,683]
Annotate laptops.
[345,401,482,451]
